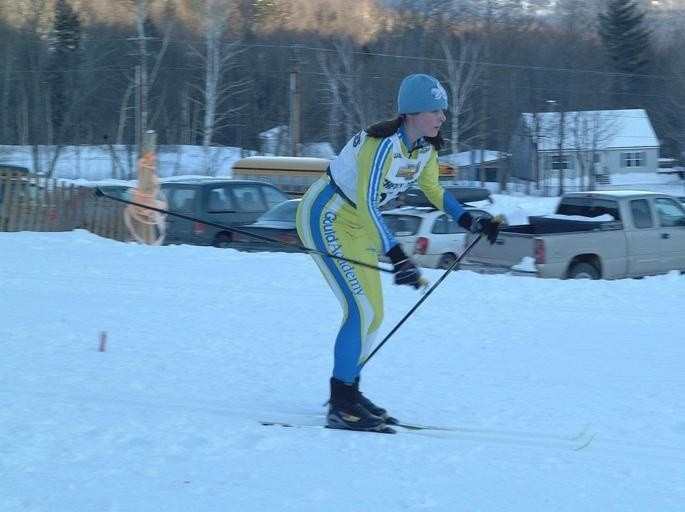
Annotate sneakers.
[327,402,388,431]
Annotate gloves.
[458,211,501,246]
[385,243,422,291]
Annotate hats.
[396,72,450,115]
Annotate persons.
[296,74,499,431]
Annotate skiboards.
[260,404,594,450]
